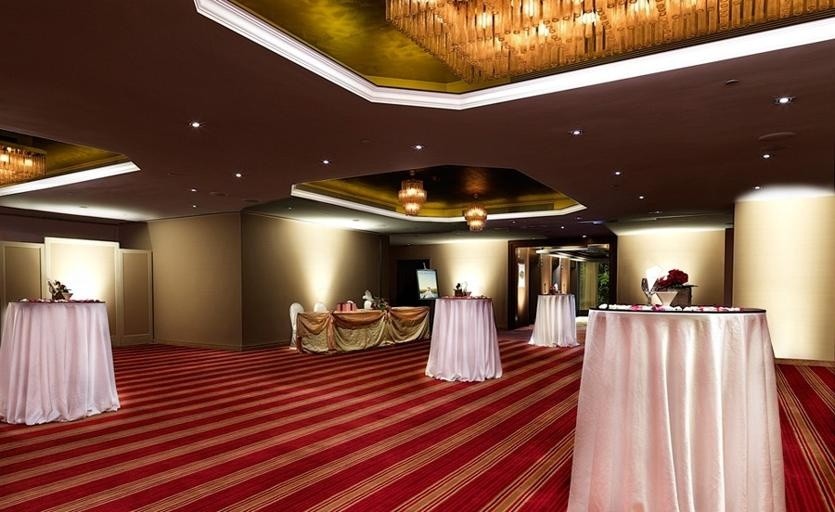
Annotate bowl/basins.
[61,292,74,300]
[655,291,679,307]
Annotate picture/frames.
[416,269,439,301]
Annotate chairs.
[288,302,328,350]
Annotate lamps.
[399,174,487,232]
[386,1,835,88]
[0,139,48,185]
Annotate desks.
[3,300,109,426]
[583,306,776,512]
[298,306,430,353]
[434,293,577,381]
